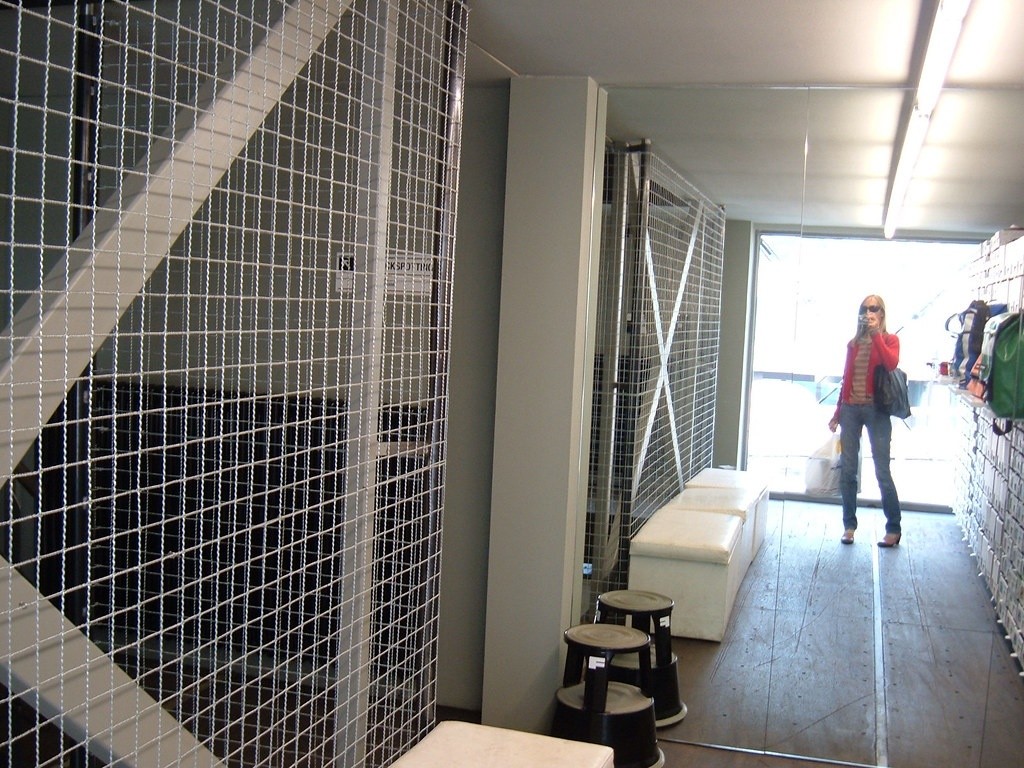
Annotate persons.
[828,294,902,548]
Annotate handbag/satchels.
[874,362,911,419]
[806,431,862,496]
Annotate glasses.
[861,305,882,314]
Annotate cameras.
[857,315,871,325]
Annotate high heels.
[878,530,902,547]
[841,528,855,543]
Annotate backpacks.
[944,299,1024,436]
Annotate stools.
[585,589,687,728]
[626,468,772,641]
[381,721,614,768]
[554,621,665,768]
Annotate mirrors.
[570,83,1024,768]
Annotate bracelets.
[869,327,880,332]
